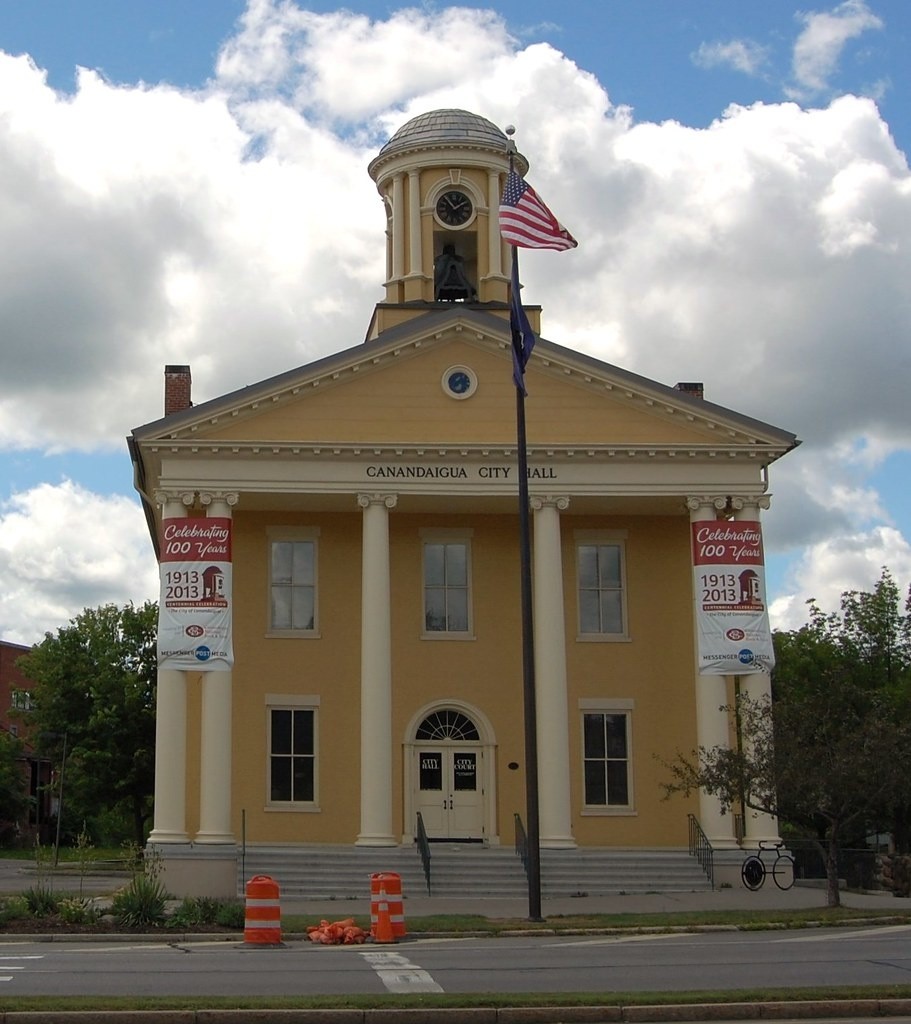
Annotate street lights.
[38,732,67,866]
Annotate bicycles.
[741,840,796,891]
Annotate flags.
[498,170,578,252]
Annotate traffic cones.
[370,881,399,944]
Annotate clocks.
[434,190,472,225]
